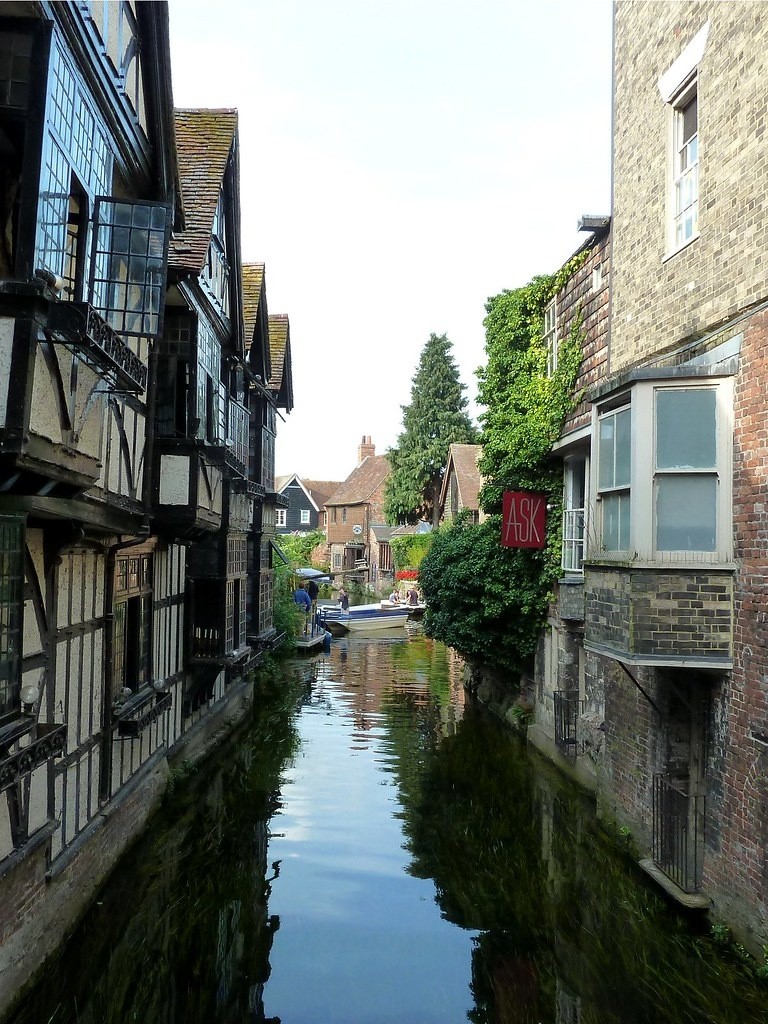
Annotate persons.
[305,581,319,620]
[337,587,349,615]
[389,586,419,606]
[293,582,311,636]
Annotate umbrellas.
[294,569,332,582]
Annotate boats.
[318,596,428,632]
